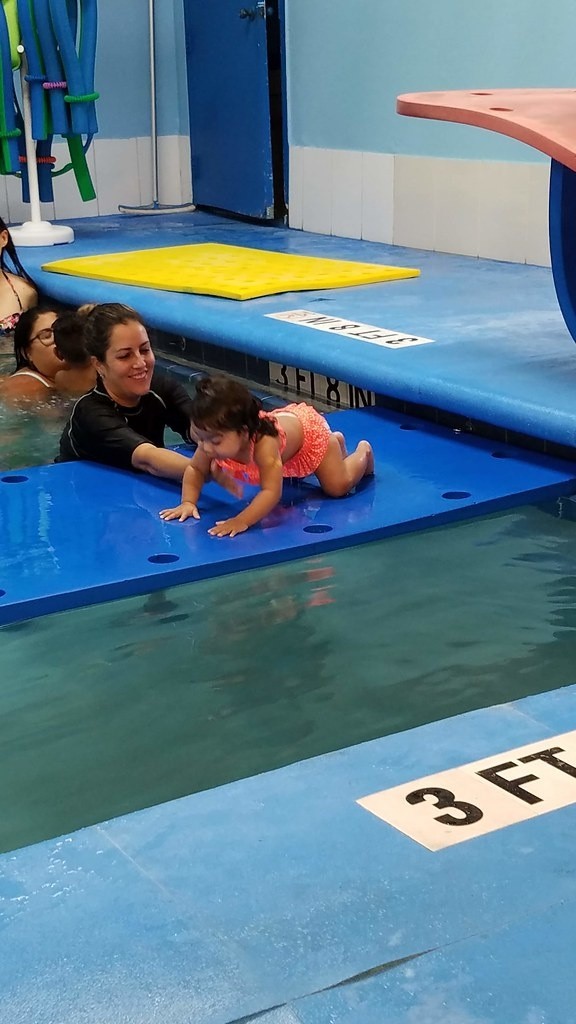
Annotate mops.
[117,0,196,215]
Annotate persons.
[158,377,374,538]
[0,217,38,335]
[1,306,68,409]
[56,303,101,394]
[56,302,248,501]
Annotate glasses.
[26,327,55,346]
